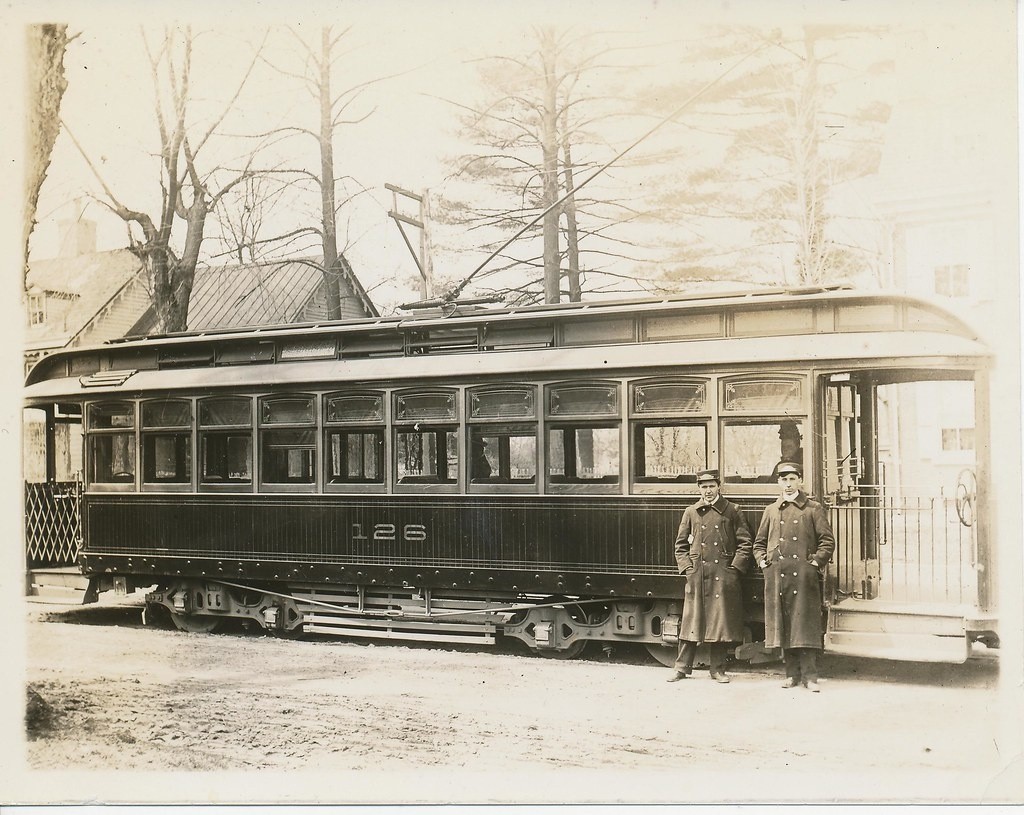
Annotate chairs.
[110,472,785,486]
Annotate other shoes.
[666,671,686,683]
[781,677,798,688]
[804,680,821,692]
[711,672,730,683]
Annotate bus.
[20,281,1001,671]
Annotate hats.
[696,469,720,483]
[778,463,801,475]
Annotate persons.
[471,437,491,478]
[768,433,803,484]
[667,469,754,683]
[753,464,835,692]
